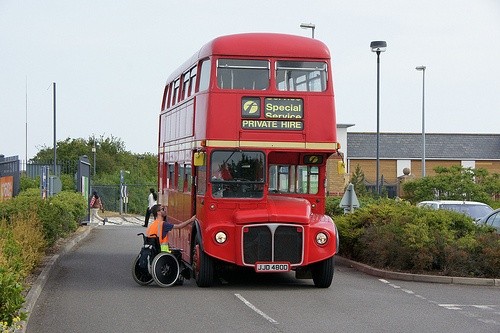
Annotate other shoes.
[103,219,105,225]
[141,225,148,227]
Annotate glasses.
[160,207,164,212]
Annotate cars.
[417,200,494,224]
[477,208,500,234]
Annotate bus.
[157,33,347,289]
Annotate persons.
[212,155,234,190]
[143,188,158,227]
[90,191,105,225]
[145,204,196,283]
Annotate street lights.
[300,23,315,38]
[91,146,96,176]
[370,40,387,194]
[415,66,428,178]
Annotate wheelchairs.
[132,233,183,288]
